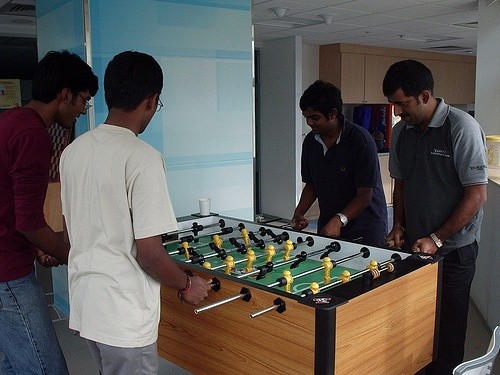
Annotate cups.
[198,199,211,216]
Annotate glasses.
[72,90,92,109]
[146,95,164,112]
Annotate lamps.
[319,14,336,25]
[269,8,288,18]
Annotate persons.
[56,49,212,375]
[291,78,389,252]
[373,59,488,375]
[0,46,98,375]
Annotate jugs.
[486,135,500,169]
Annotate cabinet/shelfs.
[380,155,396,204]
[319,43,476,104]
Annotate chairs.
[452,325,500,375]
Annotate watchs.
[430,233,444,249]
[335,212,348,226]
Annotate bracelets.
[176,275,192,303]
